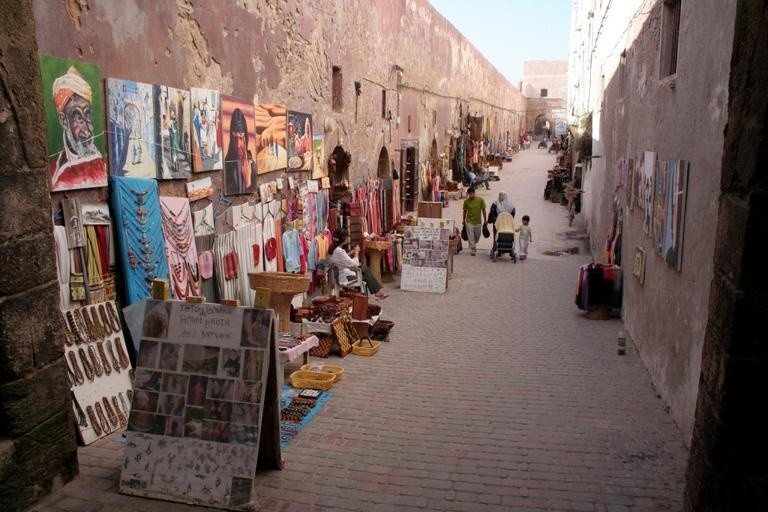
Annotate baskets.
[366,241,392,251]
[351,337,382,357]
[247,271,311,294]
[289,364,344,391]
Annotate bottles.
[616,329,626,355]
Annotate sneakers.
[519,255,526,260]
[471,247,477,256]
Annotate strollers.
[492,212,517,263]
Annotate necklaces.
[119,179,202,303]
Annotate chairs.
[321,260,367,298]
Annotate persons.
[518,129,572,153]
[516,214,532,260]
[162,111,181,171]
[127,306,270,445]
[329,227,389,299]
[466,167,491,190]
[192,96,219,161]
[223,107,258,196]
[462,188,487,256]
[489,191,515,244]
[45,65,108,190]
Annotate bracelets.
[280,398,314,442]
[58,303,136,435]
